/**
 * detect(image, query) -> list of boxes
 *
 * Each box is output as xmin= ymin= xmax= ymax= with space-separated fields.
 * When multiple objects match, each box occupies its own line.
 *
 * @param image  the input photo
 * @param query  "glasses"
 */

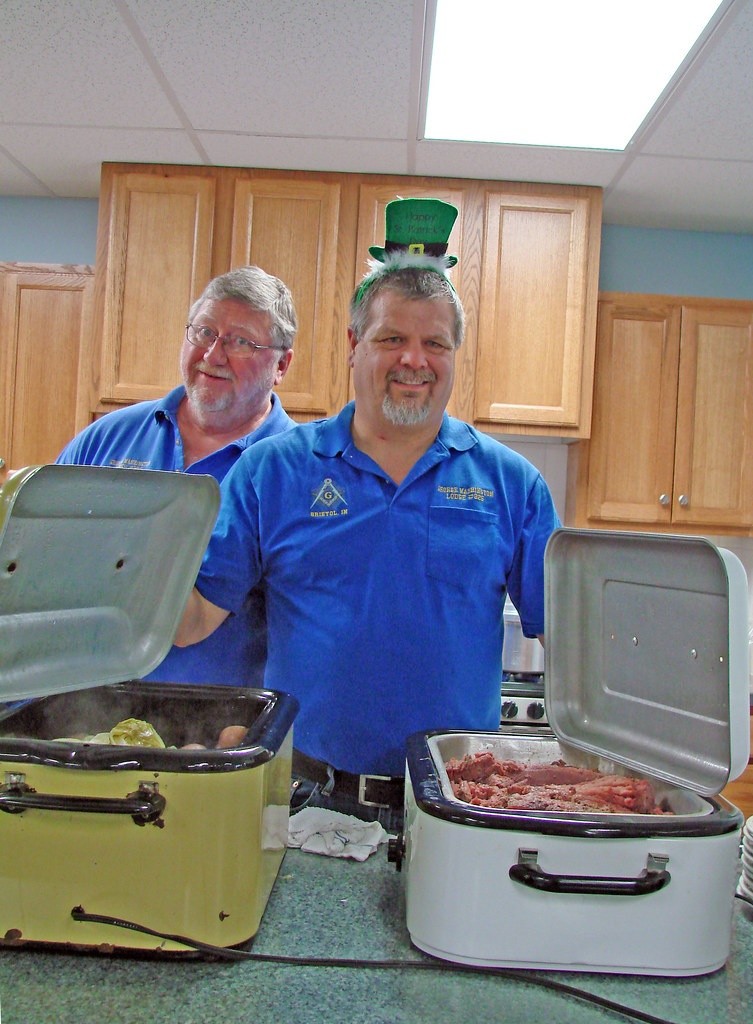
xmin=185 ymin=324 xmax=283 ymax=360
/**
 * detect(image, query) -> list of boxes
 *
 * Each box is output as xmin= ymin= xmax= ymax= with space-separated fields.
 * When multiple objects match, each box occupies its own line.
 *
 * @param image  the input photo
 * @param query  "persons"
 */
xmin=173 ymin=251 xmax=564 ymax=835
xmin=54 ymin=264 xmax=299 ymax=689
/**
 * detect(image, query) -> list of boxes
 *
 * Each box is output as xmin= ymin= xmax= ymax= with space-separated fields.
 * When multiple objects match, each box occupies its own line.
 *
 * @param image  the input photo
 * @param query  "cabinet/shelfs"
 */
xmin=0 ymin=270 xmax=95 ymax=489
xmin=350 ymin=182 xmax=465 ymax=405
xmin=227 ymin=175 xmax=345 ymax=415
xmin=472 ymin=188 xmax=591 ymax=426
xmin=98 ymin=170 xmax=219 ymax=403
xmin=563 ymin=290 xmax=753 ymax=535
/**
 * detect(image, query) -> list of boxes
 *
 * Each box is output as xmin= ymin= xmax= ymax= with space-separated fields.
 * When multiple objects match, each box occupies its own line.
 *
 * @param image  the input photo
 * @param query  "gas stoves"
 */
xmin=500 ymin=676 xmax=549 ymax=724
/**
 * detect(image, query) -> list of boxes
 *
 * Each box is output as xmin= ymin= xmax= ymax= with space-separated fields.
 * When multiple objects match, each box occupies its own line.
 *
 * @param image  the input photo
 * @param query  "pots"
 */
xmin=502 ymin=611 xmax=544 ymax=671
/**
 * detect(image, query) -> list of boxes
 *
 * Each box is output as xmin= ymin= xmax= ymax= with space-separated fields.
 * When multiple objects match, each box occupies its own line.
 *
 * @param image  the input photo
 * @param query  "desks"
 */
xmin=0 ymin=834 xmax=753 ymax=1024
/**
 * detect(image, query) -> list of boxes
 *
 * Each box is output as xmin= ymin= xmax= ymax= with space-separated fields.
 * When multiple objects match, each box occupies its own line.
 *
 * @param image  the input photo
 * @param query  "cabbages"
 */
xmin=110 ymin=718 xmax=165 ymax=748
